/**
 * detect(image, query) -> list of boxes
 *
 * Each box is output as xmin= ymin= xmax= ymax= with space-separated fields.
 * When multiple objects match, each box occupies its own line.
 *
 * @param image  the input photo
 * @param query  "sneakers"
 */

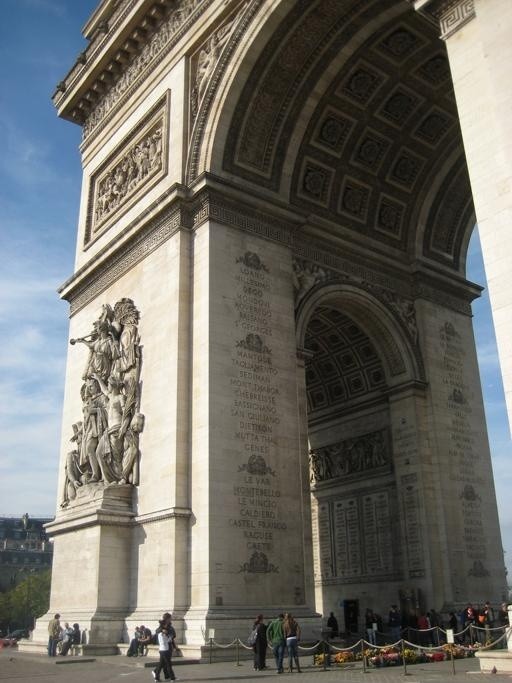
xmin=164 ymin=676 xmax=179 ymax=682
xmin=276 ymin=668 xmax=284 ymax=673
xmin=254 ymin=667 xmax=265 ymax=671
xmin=152 ymin=670 xmax=160 ymax=682
xmin=287 ymin=666 xmax=303 ymax=673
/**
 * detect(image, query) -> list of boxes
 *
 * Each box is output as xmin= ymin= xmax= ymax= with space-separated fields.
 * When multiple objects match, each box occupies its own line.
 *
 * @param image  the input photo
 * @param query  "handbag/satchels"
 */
xmin=478 ymin=616 xmax=485 ymax=623
xmin=373 ymin=623 xmax=378 ymax=631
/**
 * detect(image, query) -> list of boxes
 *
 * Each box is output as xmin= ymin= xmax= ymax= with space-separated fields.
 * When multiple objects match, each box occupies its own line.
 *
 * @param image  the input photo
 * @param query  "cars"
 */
xmin=7 ymin=630 xmax=25 ymax=640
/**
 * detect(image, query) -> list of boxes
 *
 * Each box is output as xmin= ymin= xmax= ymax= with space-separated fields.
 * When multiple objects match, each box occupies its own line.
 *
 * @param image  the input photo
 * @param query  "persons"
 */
xmin=137 ymin=624 xmax=152 ymax=656
xmin=67 ymin=396 xmax=109 ymax=483
xmin=404 ymin=600 xmax=512 ymax=644
xmin=126 ymin=625 xmax=143 ymax=655
xmin=326 ymin=610 xmax=337 ymax=639
xmin=151 ymin=611 xmax=177 ymax=679
xmin=366 ymin=607 xmax=384 ymax=635
xmin=282 ymin=612 xmax=305 ymax=672
xmin=76 ymin=321 xmax=117 ymax=392
xmin=88 ymin=367 xmax=131 ymax=484
xmin=59 ymin=622 xmax=80 ymax=655
xmin=153 ymin=619 xmax=180 ymax=682
xmin=265 ymin=612 xmax=288 ymax=673
xmin=248 ymin=613 xmax=271 ymax=670
xmin=47 ymin=612 xmax=61 ymax=655
xmin=388 ymin=603 xmax=402 ymax=640
xmin=57 ymin=621 xmax=73 ymax=654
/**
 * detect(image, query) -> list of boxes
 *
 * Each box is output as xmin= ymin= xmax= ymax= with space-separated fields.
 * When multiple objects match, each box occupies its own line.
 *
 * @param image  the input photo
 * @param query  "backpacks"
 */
xmin=246 ymin=624 xmax=261 ymax=647
